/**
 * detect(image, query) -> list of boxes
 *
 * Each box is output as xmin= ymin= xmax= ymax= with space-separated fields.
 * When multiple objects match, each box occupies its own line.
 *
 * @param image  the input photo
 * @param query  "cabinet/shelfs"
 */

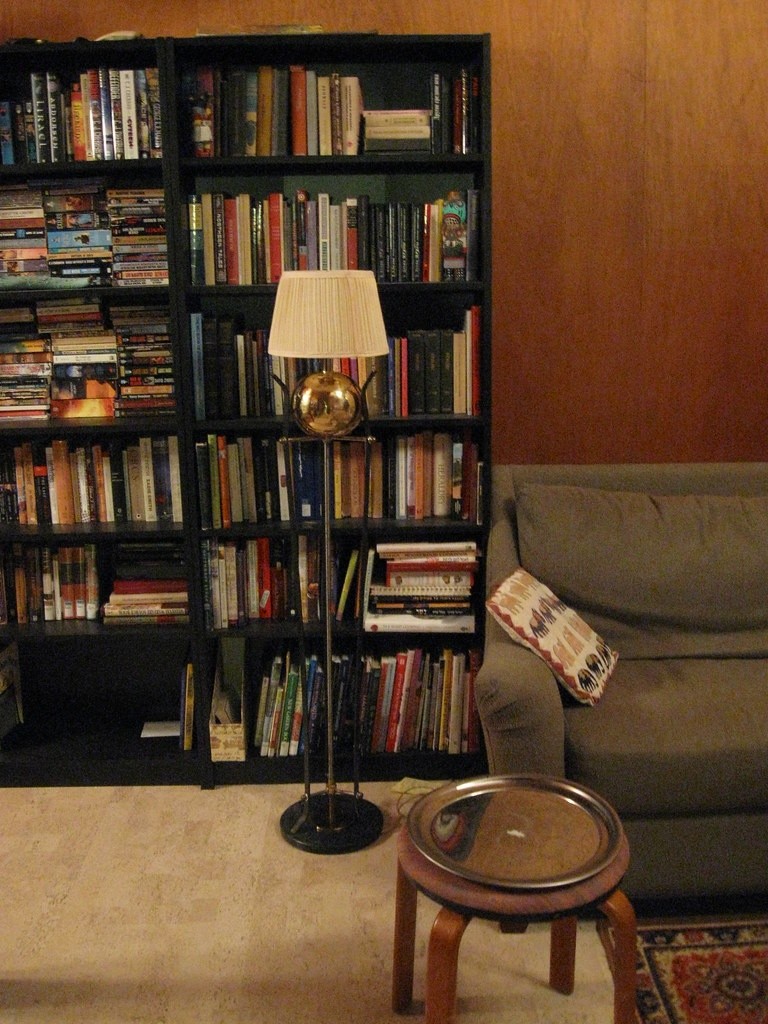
xmin=0 ymin=36 xmax=198 ymax=789
xmin=165 ymin=29 xmax=493 ymax=789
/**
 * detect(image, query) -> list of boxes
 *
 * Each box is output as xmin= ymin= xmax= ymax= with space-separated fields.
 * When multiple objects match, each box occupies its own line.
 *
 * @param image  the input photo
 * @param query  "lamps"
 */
xmin=265 ymin=268 xmax=391 ymax=856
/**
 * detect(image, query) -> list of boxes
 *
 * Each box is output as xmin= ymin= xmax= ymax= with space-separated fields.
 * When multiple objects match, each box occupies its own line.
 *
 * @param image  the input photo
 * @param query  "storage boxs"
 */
xmin=208 ymin=639 xmax=246 ymax=762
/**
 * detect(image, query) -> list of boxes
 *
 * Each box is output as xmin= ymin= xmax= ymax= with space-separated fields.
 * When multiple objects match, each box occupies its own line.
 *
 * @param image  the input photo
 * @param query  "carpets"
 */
xmin=595 ymin=911 xmax=768 ymax=1024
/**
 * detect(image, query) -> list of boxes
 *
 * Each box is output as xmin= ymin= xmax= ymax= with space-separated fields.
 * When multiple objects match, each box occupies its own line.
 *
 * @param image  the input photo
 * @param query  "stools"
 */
xmin=390 ymin=774 xmax=638 ymax=1024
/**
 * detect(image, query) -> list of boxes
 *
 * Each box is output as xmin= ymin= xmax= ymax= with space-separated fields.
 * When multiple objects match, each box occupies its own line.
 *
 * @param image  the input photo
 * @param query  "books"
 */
xmin=1 ymin=642 xmax=481 ymax=759
xmin=0 ymin=66 xmax=480 ymax=633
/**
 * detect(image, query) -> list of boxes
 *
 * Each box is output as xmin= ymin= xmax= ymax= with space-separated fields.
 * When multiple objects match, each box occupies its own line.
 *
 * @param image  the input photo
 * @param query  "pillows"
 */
xmin=489 ymin=566 xmax=619 ymax=705
xmin=514 ymin=482 xmax=768 ymax=657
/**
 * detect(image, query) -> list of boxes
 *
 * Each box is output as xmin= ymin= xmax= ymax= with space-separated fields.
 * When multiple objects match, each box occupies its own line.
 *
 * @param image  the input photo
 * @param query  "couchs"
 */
xmin=475 ymin=465 xmax=768 ymax=930
